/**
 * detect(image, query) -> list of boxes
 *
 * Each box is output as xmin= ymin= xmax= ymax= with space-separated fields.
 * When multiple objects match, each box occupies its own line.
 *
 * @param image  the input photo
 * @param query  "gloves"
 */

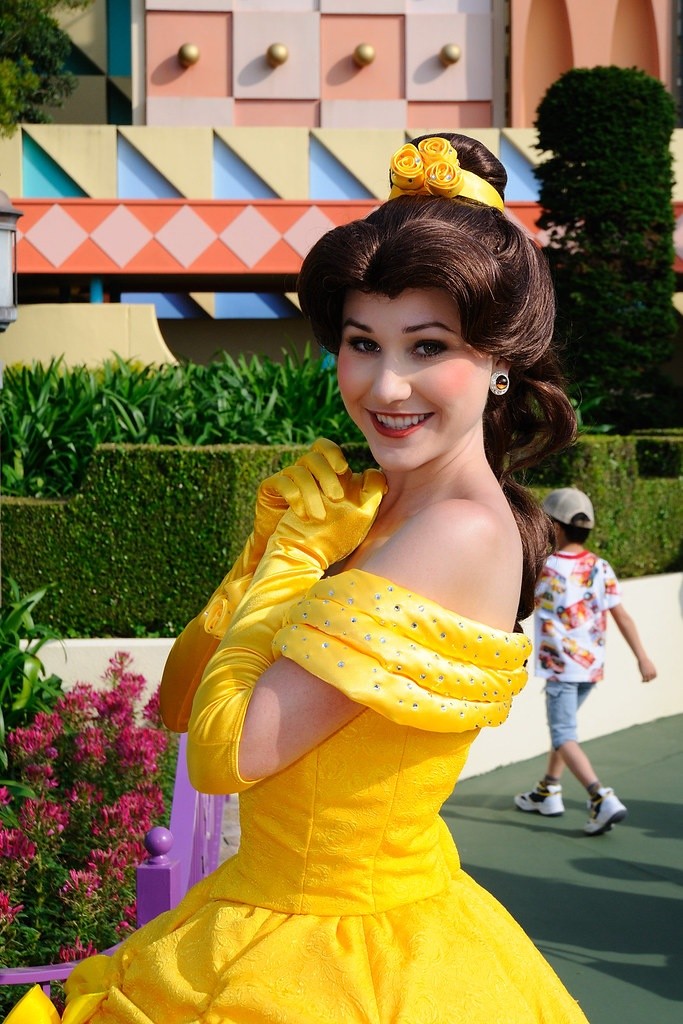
xmin=189 ymin=465 xmax=387 ymax=794
xmin=158 ymin=435 xmax=348 ymax=730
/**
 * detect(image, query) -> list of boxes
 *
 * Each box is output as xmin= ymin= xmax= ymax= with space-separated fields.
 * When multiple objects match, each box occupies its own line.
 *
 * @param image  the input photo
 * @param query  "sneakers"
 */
xmin=512 ymin=781 xmax=566 ymax=816
xmin=583 ymin=786 xmax=627 ymax=836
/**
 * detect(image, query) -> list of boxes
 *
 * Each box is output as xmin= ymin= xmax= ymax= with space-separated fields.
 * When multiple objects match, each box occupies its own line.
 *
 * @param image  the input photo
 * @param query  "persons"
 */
xmin=514 ymin=484 xmax=656 ymax=831
xmin=1 ymin=133 xmax=589 ymax=1024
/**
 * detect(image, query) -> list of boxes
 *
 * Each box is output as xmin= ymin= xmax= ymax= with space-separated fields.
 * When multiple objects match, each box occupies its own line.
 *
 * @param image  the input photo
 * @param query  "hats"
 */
xmin=539 ymin=484 xmax=595 ymax=530
